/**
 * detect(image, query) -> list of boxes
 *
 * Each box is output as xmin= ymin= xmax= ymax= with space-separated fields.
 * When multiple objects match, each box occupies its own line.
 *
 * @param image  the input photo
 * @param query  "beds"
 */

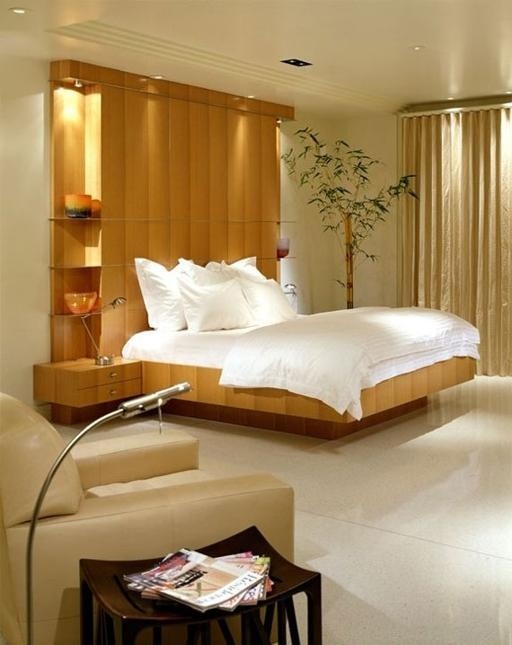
xmin=121 ymin=303 xmax=480 ymax=442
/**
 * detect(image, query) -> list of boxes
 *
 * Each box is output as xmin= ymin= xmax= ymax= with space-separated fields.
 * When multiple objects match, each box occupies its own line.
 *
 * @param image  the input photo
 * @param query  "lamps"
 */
xmin=80 ymin=296 xmax=129 ymax=366
xmin=25 ymin=382 xmax=193 ymax=645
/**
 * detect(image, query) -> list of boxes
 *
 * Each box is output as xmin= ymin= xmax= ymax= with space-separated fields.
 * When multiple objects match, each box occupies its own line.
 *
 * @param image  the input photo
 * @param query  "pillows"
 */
xmin=133 ymin=252 xmax=297 ymax=332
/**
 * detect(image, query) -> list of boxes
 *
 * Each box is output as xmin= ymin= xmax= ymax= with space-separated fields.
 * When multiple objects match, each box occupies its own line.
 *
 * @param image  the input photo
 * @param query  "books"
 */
xmin=121 ymin=546 xmax=276 ymax=614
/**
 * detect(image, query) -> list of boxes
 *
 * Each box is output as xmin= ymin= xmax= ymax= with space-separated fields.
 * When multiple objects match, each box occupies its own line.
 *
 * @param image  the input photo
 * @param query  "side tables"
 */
xmin=76 ymin=524 xmax=321 ymax=645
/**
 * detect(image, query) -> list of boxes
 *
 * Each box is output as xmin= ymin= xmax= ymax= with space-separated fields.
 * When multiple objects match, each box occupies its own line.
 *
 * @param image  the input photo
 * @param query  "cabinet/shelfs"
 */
xmin=51 ymin=215 xmax=102 ymax=318
xmin=277 ymin=219 xmax=299 ymax=295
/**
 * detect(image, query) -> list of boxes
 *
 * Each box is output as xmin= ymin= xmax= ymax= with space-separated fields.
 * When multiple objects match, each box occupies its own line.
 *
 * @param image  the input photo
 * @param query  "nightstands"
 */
xmin=33 ymin=357 xmax=143 ymax=425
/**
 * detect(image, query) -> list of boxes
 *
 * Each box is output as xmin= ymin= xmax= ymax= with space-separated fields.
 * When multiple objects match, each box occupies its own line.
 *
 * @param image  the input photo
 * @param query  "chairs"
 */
xmin=0 ymin=392 xmax=297 ymax=645
